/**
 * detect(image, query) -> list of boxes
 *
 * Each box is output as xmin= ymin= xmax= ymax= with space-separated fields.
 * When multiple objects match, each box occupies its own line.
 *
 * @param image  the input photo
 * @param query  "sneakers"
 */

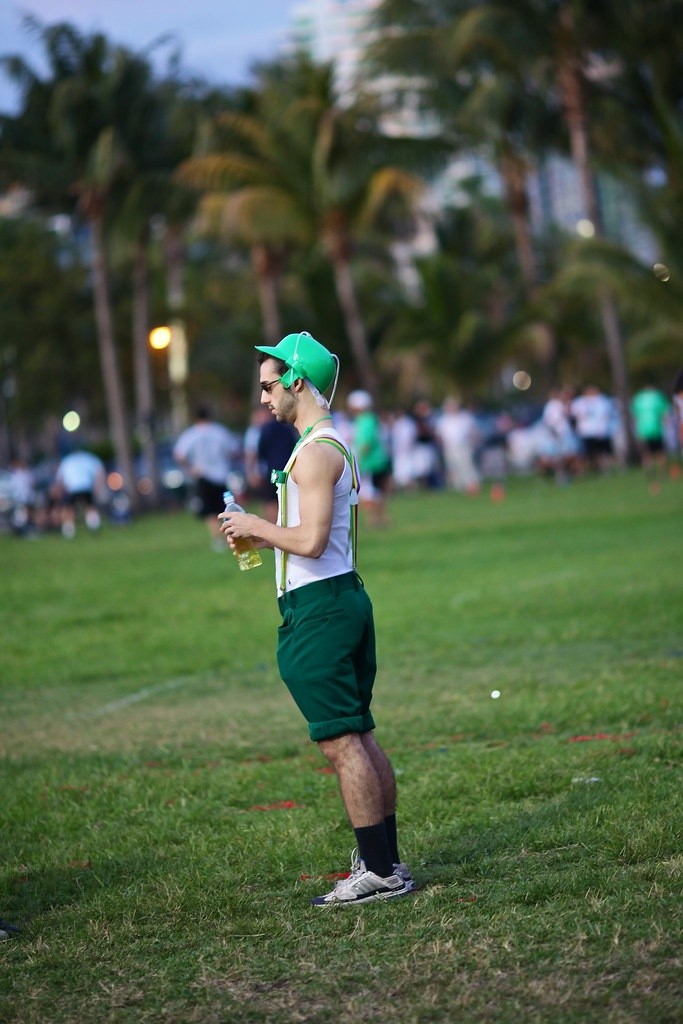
xmin=312 ymin=847 xmax=417 ymax=908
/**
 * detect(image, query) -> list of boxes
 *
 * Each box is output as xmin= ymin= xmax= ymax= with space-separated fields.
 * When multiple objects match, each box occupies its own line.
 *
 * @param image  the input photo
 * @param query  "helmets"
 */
xmin=254 ymin=333 xmax=337 ymax=395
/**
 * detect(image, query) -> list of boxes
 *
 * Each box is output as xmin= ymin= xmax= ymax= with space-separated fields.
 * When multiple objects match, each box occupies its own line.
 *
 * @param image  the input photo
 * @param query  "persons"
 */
xmin=0 ymin=374 xmax=683 ymax=540
xmin=218 ymin=332 xmax=413 ymax=909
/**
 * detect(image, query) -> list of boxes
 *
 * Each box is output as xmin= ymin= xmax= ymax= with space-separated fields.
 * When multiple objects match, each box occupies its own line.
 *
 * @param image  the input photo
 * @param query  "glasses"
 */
xmin=260 ymin=380 xmax=281 ymax=393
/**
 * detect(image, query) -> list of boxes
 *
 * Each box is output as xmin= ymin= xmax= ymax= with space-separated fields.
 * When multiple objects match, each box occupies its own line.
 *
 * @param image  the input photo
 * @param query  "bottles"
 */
xmin=222 ymin=491 xmax=262 ymax=571
